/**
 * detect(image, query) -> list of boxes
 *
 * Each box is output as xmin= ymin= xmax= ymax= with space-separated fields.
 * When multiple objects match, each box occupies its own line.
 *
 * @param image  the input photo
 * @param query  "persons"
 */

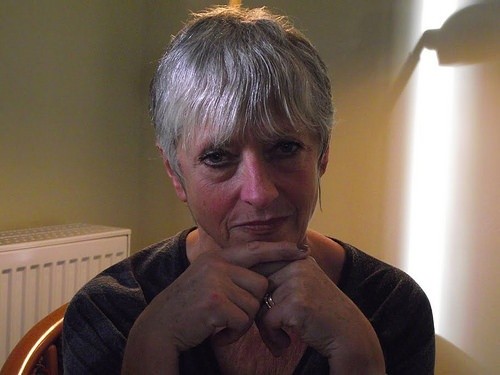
xmin=57 ymin=2 xmax=437 ymax=375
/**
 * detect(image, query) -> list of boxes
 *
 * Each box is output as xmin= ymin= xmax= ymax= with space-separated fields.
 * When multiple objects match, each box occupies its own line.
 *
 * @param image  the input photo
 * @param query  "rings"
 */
xmin=264 ymin=293 xmax=275 ymax=310
